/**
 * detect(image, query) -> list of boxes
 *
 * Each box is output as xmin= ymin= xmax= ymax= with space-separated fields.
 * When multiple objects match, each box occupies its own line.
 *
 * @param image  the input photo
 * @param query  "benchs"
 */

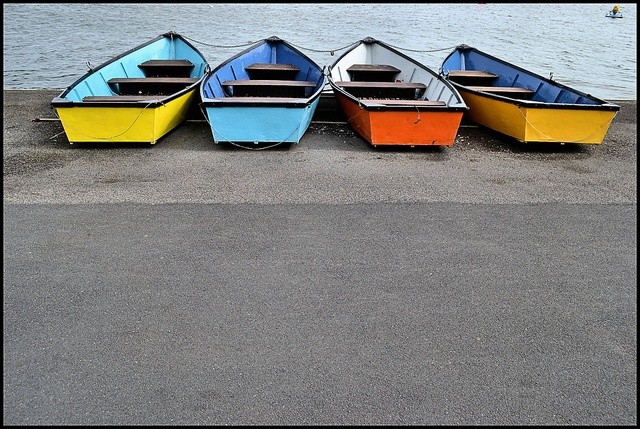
xmin=211 ymin=97 xmax=308 ymax=102
xmin=244 ymin=63 xmax=301 ymax=71
xmin=346 ymin=64 xmax=401 ymax=72
xmin=448 ymin=70 xmax=498 ymax=78
xmin=360 ymin=99 xmax=445 ymax=105
xmin=138 ymin=59 xmax=194 ymax=66
xmin=221 ymin=80 xmax=317 ymax=87
xmin=107 ymin=78 xmax=201 ymax=84
xmin=82 ymin=96 xmax=169 ymax=101
xmin=464 ymin=86 xmax=535 ymax=94
xmin=335 ymin=81 xmax=427 ymax=89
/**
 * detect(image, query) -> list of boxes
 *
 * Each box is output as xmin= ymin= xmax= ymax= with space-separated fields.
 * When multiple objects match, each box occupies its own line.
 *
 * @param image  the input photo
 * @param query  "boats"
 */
xmin=49 ymin=29 xmax=210 ymax=144
xmin=439 ymin=42 xmax=620 ymax=146
xmin=199 ymin=35 xmax=326 ymax=151
xmin=326 ymin=37 xmax=466 ymax=152
